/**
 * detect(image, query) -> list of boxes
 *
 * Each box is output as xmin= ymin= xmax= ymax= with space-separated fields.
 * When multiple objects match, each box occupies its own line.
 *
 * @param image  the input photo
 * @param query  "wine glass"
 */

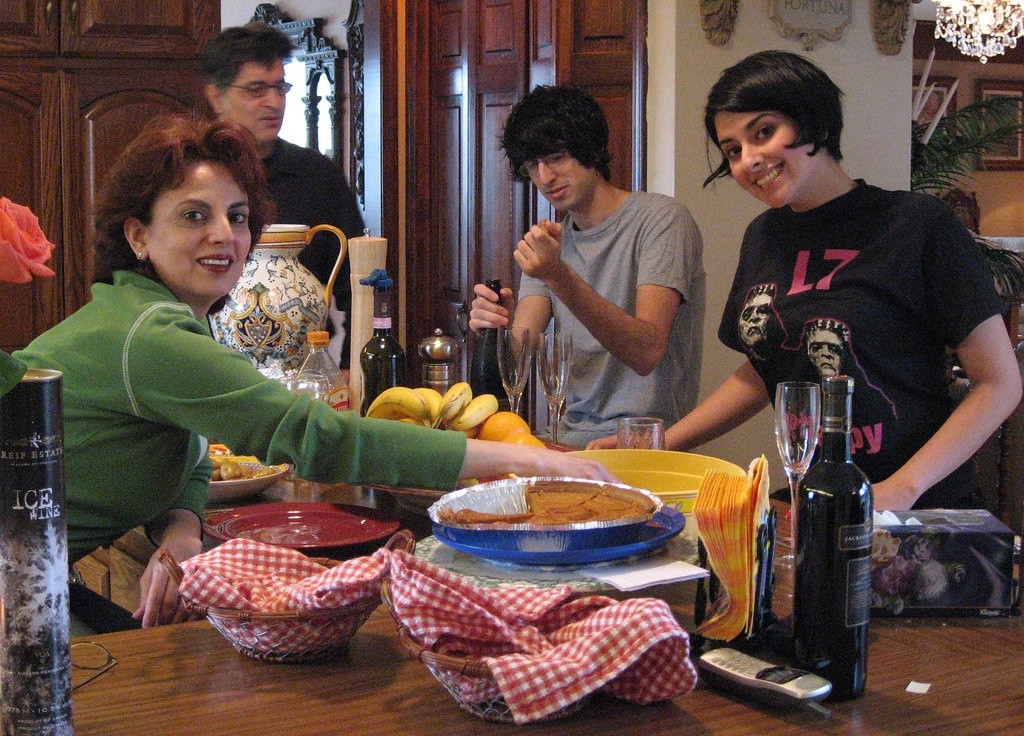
xmin=773 ymin=381 xmax=821 ymax=569
xmin=536 ymin=331 xmax=573 ymax=450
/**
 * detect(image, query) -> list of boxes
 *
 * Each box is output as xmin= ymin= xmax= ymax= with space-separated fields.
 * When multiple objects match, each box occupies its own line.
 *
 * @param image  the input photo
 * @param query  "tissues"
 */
xmin=867 ymin=506 xmax=1023 ymax=618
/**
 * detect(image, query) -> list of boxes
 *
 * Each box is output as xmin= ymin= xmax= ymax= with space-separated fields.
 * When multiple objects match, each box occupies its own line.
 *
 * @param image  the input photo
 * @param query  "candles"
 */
xmin=921 ymin=78 xmax=962 ymax=144
xmin=916 ymin=83 xmax=935 ymax=121
xmin=913 ymin=45 xmax=935 ymax=121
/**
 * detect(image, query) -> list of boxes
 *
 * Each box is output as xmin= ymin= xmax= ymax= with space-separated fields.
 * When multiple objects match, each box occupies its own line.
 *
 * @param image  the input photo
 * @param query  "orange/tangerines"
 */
xmin=480 ymin=412 xmax=547 ymax=449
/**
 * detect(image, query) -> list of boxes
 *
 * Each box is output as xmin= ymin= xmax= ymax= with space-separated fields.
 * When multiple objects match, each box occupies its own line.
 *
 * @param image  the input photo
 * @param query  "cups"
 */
xmin=617 ymin=417 xmax=665 ymax=450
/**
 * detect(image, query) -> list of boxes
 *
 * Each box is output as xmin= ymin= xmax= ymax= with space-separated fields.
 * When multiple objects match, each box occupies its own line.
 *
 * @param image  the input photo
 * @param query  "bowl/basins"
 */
xmin=201 ymin=455 xmax=290 ymax=501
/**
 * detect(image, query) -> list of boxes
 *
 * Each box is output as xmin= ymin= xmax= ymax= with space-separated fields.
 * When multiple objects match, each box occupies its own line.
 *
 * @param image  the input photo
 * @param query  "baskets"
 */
xmin=378 ymin=578 xmax=596 ymax=723
xmin=159 ymin=528 xmax=417 ymax=664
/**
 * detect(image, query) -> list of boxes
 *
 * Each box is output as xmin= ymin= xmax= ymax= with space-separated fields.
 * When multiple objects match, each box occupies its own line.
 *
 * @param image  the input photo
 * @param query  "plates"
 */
xmin=202 ymin=502 xmax=401 ymax=550
xmin=432 ymin=505 xmax=687 ymax=566
xmin=552 ymin=449 xmax=747 ymax=517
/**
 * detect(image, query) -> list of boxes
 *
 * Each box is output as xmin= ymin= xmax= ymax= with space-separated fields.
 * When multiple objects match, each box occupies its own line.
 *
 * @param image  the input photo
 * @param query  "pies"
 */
xmin=434 ymin=481 xmax=654 ymax=524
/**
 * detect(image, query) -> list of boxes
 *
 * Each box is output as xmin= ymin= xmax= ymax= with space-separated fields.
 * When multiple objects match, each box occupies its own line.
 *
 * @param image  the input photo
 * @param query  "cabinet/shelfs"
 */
xmin=0 ymin=0 xmax=222 ymax=348
xmin=379 ymin=0 xmax=645 ymax=403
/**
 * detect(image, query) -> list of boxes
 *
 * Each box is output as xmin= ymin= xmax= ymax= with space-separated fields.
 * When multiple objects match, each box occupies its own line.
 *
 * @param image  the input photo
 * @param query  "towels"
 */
xmin=177 ymin=539 xmax=390 ymax=612
xmin=390 ymin=547 xmax=697 ymax=725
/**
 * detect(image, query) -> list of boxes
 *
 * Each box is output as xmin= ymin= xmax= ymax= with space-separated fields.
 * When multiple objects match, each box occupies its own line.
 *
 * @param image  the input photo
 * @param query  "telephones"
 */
xmin=698 ymin=646 xmax=834 ymax=718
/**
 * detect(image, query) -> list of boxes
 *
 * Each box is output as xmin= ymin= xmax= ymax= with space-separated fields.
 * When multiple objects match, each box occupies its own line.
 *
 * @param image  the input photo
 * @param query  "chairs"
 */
xmin=944 ymin=294 xmax=1024 ymax=533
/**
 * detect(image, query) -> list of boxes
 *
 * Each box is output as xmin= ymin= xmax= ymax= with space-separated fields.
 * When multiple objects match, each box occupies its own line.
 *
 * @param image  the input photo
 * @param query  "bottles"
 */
xmin=790 ymin=374 xmax=874 ymax=703
xmin=0 ymin=368 xmax=75 ymax=736
xmin=496 ymin=326 xmax=532 ymax=415
xmin=417 ymin=327 xmax=461 ymax=397
xmin=469 ymin=279 xmax=524 ymax=413
xmin=293 ymin=330 xmax=352 ymax=500
xmin=359 ymin=268 xmax=406 ymax=498
xmin=207 ymin=222 xmax=347 ymax=382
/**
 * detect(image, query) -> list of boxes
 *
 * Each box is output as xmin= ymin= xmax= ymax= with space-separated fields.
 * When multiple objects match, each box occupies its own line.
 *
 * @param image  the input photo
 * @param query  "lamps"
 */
xmin=933 ymin=0 xmax=1024 ymax=63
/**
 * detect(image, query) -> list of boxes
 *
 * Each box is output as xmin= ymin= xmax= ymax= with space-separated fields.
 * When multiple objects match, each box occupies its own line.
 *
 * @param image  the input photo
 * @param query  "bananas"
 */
xmin=366 ymin=382 xmax=499 ymax=440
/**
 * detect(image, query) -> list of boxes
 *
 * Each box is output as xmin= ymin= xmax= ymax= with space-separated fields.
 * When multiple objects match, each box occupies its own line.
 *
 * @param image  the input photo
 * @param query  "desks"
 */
xmin=68 ymin=432 xmax=1024 ymax=736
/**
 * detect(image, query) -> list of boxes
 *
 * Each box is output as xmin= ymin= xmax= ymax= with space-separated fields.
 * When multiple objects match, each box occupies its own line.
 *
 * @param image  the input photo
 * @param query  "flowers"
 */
xmin=0 ymin=195 xmax=55 ymax=386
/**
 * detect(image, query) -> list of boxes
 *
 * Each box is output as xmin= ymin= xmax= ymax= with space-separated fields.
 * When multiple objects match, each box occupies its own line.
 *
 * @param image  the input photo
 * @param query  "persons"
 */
xmin=10 ymin=115 xmax=617 ymax=639
xmin=201 ymin=24 xmax=371 ymax=370
xmin=586 ymin=51 xmax=1022 ymax=512
xmin=468 ymin=84 xmax=705 ymax=450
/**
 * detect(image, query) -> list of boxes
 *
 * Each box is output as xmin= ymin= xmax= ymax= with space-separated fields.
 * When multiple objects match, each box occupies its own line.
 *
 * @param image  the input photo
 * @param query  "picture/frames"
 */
xmin=974 ymin=80 xmax=1024 ymax=172
xmin=909 ymin=76 xmax=958 ymax=166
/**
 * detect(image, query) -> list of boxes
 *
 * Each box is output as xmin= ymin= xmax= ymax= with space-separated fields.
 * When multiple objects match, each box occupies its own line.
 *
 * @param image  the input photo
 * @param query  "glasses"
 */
xmin=227 ymin=82 xmax=293 ymax=97
xmin=515 ymin=152 xmax=571 ymax=178
